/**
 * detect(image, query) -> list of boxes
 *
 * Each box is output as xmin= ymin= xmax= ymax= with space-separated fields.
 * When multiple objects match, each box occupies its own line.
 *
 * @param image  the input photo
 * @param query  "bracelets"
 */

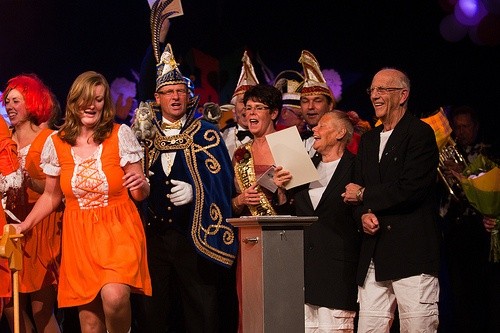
xmin=233 ymin=199 xmax=241 ymax=211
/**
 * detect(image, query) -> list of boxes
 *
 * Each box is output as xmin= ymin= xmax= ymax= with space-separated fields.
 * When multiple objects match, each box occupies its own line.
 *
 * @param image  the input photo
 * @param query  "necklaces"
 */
xmin=305 ymin=139 xmax=316 ymax=154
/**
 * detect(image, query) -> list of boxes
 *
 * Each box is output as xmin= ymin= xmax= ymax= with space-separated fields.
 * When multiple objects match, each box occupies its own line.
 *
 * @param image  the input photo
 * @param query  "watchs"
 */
xmin=356 ymin=187 xmax=365 ymax=203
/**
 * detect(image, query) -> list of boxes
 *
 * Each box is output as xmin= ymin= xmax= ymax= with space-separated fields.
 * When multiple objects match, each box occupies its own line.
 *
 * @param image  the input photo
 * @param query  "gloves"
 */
xmin=169 ymin=179 xmax=193 ymax=206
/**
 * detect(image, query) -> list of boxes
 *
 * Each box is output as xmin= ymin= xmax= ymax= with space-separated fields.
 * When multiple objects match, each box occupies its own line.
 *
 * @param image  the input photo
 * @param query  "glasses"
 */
xmin=366 ymin=87 xmax=403 ymax=95
xmin=242 ymin=105 xmax=271 ymax=113
xmin=288 ymin=108 xmax=303 ymax=119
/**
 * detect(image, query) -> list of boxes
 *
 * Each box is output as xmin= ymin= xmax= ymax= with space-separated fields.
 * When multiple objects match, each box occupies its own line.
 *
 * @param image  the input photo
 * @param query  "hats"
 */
xmin=273 ymin=70 xmax=306 ymax=108
xmin=230 ymin=51 xmax=260 ymax=103
xmin=298 ymin=50 xmax=337 ymax=104
xmin=156 ymin=43 xmax=187 ymax=91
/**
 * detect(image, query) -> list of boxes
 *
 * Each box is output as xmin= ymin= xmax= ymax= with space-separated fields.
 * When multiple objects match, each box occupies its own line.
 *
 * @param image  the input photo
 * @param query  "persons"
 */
xmin=341 ymin=67 xmax=442 ymax=333
xmin=3 ymin=70 xmax=151 ymax=333
xmin=129 ymin=76 xmax=240 ymax=333
xmin=2 ymin=73 xmax=65 ymax=333
xmin=218 ymin=79 xmax=371 ymax=161
xmin=0 ymin=113 xmax=36 ymax=333
xmin=482 ymin=215 xmax=497 ymax=233
xmin=231 ymin=85 xmax=293 ymax=333
xmin=272 ymin=109 xmax=363 ymax=333
xmin=441 ymin=109 xmax=500 ymax=333
